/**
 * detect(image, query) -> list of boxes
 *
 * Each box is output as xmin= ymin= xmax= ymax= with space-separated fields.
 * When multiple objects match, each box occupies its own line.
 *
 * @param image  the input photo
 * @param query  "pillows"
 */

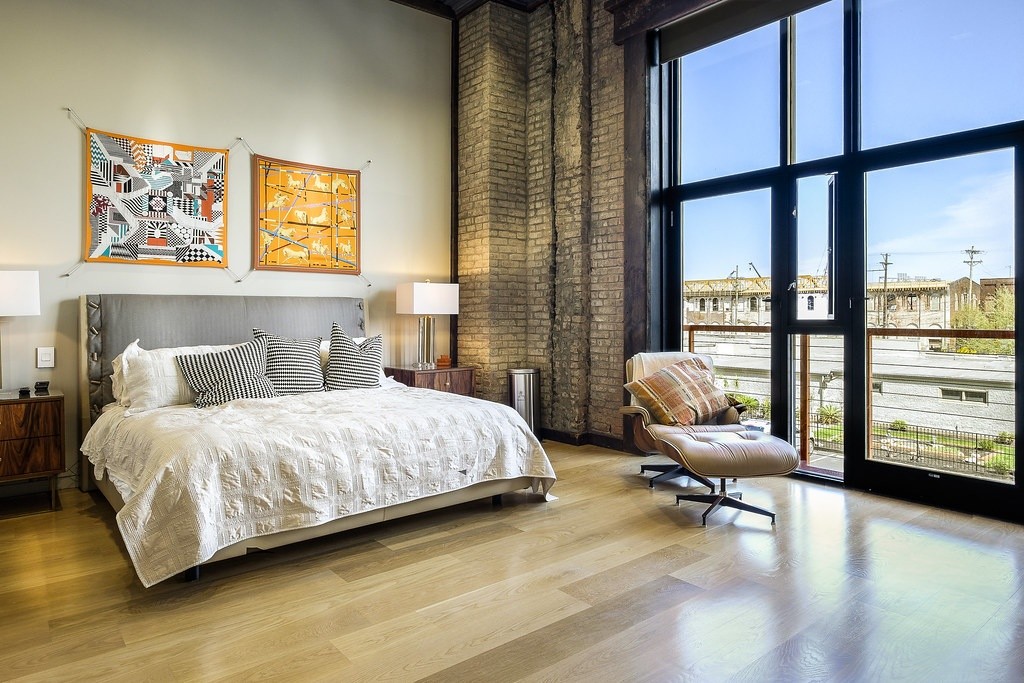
xmin=175 ymin=336 xmax=281 ymax=408
xmin=622 ymin=357 xmax=729 ymax=428
xmin=252 ymin=327 xmax=325 ymax=397
xmin=320 ymin=337 xmax=388 ymax=381
xmin=121 ymin=338 xmax=250 ymax=417
xmin=324 ymin=321 xmax=383 ymax=391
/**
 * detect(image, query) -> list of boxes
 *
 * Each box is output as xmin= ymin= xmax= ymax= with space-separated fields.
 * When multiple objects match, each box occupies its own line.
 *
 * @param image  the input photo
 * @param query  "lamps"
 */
xmin=395 ymin=279 xmax=459 ymax=367
xmin=0 ymin=270 xmax=41 ymax=390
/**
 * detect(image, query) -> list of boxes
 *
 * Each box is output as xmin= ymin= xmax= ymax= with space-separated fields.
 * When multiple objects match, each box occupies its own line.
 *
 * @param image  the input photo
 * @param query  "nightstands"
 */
xmin=0 ymin=389 xmax=66 ymax=515
xmin=385 ymin=367 xmax=476 ymax=398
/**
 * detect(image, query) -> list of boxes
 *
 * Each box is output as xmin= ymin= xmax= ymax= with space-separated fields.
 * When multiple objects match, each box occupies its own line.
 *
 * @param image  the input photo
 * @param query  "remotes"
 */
xmin=18 ymin=387 xmax=30 ymax=393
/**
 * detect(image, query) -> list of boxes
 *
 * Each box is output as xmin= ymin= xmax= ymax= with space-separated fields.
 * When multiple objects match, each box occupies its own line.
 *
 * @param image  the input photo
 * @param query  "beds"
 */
xmin=78 ymin=295 xmax=560 ymax=585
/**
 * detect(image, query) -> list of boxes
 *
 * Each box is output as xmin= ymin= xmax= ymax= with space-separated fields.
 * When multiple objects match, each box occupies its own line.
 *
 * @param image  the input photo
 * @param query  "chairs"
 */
xmin=619 ymin=351 xmax=800 ymax=527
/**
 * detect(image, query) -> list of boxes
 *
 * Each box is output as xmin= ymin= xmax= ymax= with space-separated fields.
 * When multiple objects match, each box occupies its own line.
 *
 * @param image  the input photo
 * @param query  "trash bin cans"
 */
xmin=507 ymin=367 xmax=542 ymax=441
